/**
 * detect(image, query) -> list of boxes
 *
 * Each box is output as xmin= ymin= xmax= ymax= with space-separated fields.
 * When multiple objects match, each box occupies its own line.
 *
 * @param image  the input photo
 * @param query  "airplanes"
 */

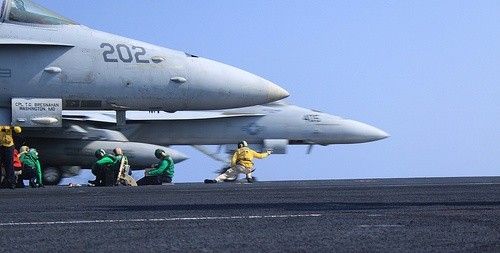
xmin=62 ymin=102 xmax=390 ymax=182
xmin=0 ymin=0 xmax=290 ymax=188
xmin=16 ymin=120 xmax=189 ymax=184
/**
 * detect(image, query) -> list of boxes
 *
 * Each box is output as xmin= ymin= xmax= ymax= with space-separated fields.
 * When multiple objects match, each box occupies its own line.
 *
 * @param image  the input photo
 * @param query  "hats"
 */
xmin=19 ymin=144 xmax=30 ymax=154
xmin=152 ymin=148 xmax=165 ymax=158
xmin=112 ymin=147 xmax=125 ymax=155
xmin=93 ymin=149 xmax=106 ymax=159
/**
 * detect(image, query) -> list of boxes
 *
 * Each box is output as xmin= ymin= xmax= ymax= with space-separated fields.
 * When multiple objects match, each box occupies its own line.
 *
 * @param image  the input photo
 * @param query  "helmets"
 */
xmin=236 ymin=140 xmax=250 ymax=149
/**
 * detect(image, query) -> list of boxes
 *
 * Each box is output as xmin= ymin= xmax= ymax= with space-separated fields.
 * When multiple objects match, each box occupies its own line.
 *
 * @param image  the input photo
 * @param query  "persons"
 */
xmin=204 ymin=140 xmax=274 ymax=183
xmin=0 ymin=146 xmax=45 ymax=189
xmin=87 ymin=147 xmax=133 ymax=186
xmin=136 ymin=149 xmax=175 ymax=186
xmin=0 ymin=126 xmax=22 ymax=190
